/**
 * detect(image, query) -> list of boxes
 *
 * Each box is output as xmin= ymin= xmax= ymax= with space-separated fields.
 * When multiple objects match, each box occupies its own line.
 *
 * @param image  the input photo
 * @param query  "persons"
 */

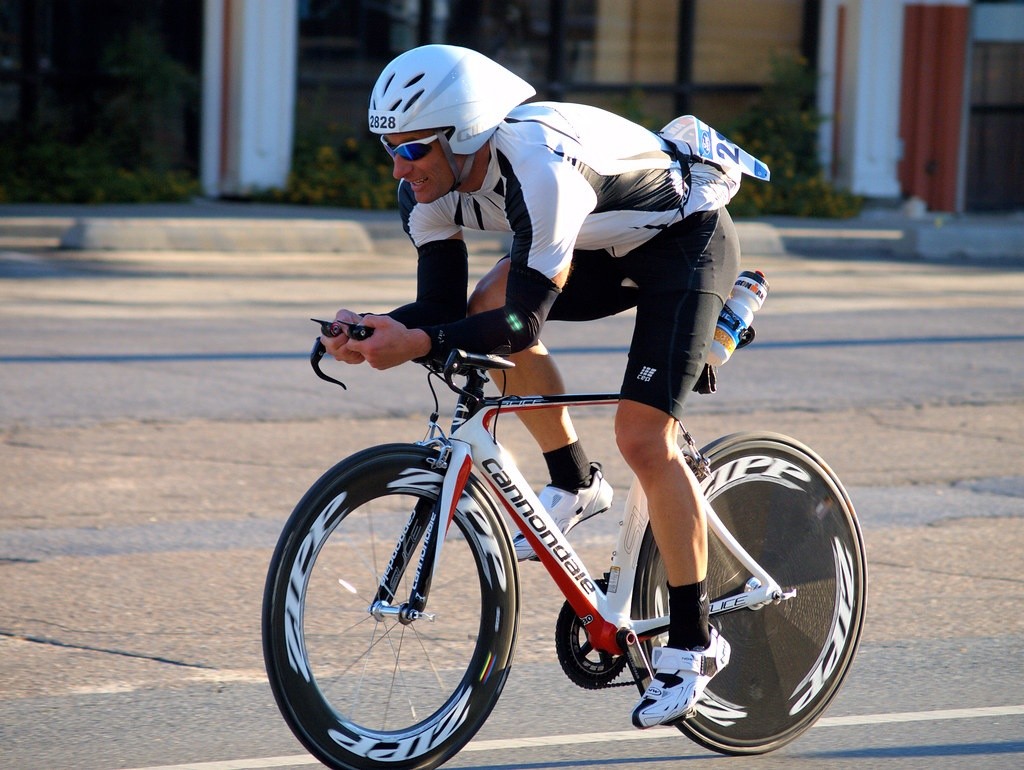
xmin=321 ymin=46 xmax=742 ymax=728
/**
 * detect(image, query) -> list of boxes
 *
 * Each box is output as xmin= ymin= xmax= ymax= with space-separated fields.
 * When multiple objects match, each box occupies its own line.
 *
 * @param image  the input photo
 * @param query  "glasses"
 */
xmin=381 ymin=129 xmax=453 ymax=162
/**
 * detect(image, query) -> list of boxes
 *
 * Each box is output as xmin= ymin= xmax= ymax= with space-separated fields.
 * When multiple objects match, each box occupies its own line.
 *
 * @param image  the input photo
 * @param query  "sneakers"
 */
xmin=512 ymin=462 xmax=613 ymax=562
xmin=630 ymin=623 xmax=731 ymax=729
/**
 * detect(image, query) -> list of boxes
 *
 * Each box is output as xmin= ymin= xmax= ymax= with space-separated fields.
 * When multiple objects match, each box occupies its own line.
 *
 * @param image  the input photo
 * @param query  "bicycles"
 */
xmin=263 ymin=316 xmax=869 ymax=770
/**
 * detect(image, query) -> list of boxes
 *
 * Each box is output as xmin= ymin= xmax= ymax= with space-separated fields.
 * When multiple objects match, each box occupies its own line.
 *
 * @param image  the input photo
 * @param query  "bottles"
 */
xmin=704 ymin=269 xmax=770 ymax=368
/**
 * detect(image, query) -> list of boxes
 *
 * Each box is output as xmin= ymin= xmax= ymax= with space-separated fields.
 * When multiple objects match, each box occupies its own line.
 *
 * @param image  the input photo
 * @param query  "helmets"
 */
xmin=368 ymin=44 xmax=536 ymax=156
